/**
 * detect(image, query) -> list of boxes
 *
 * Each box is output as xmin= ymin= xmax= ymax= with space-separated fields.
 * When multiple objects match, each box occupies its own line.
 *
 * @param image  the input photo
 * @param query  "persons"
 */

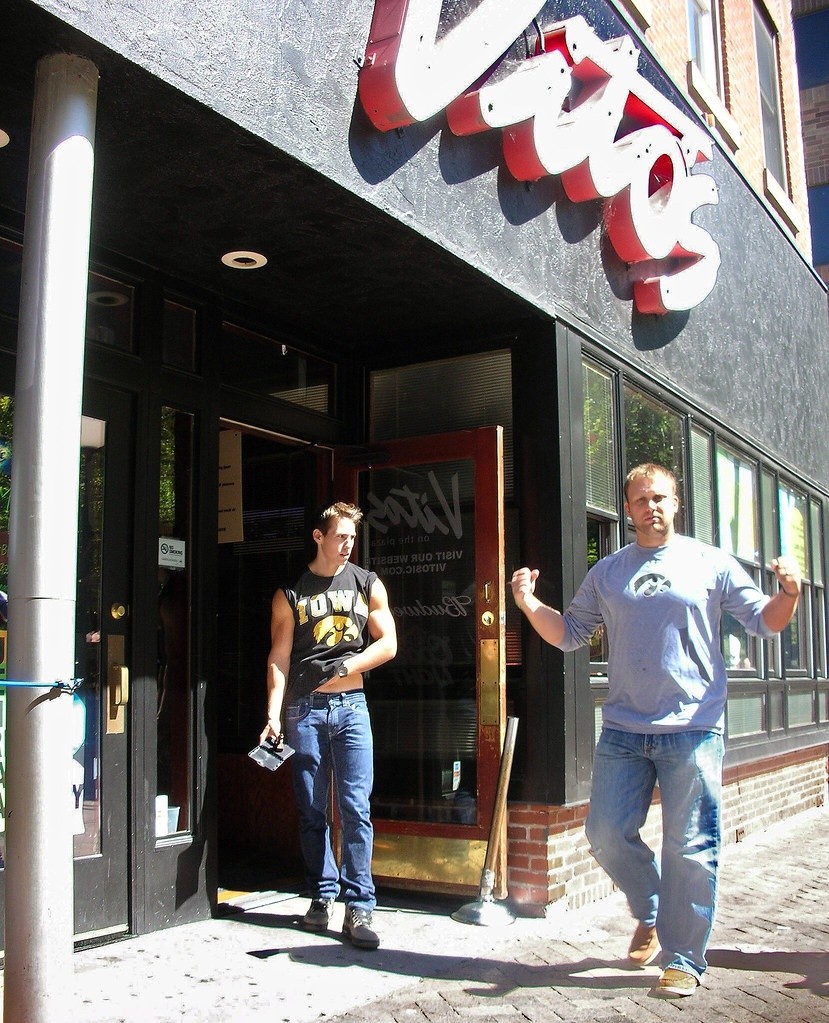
xmin=512 ymin=464 xmax=801 ymax=995
xmin=157 ymin=545 xmax=187 ymax=830
xmin=259 ymin=501 xmax=397 ymax=947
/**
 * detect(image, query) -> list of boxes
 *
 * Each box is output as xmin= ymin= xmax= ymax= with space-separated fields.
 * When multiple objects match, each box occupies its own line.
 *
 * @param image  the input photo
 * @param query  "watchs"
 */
xmin=338 ymin=662 xmax=348 ymax=678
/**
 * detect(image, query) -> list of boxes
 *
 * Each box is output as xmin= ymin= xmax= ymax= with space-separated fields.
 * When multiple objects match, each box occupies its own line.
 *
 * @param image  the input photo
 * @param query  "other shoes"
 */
xmin=303 ymin=898 xmax=333 ymax=931
xmin=656 ymin=969 xmax=697 ymax=995
xmin=343 ymin=907 xmax=379 ymax=949
xmin=630 ymin=927 xmax=660 ymax=966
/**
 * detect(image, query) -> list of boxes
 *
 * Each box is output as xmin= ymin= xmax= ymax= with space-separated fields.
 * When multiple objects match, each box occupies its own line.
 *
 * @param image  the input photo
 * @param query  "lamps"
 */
xmin=220 ymin=248 xmax=269 ymax=270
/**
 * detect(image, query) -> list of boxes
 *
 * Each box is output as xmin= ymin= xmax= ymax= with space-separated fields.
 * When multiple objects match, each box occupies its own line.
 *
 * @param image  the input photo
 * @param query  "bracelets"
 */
xmin=783 ymin=588 xmax=800 ymax=596
